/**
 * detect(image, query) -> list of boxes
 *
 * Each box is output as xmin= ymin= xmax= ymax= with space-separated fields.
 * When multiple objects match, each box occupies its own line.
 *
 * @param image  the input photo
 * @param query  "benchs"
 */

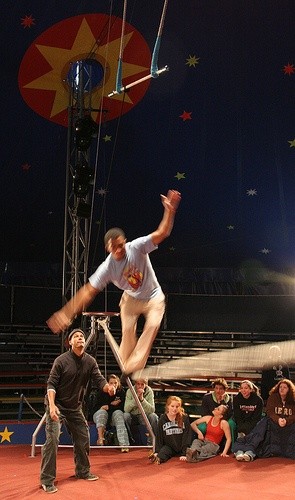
xmin=0 ymin=324 xmax=295 ymax=419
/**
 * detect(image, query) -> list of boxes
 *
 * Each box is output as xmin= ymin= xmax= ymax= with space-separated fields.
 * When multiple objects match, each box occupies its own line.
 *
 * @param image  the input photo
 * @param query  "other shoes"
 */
xmin=75 ymin=473 xmax=99 ymax=480
xmin=41 ymin=483 xmax=58 ymax=493
xmin=120 ymin=448 xmax=129 ymax=453
xmin=96 ymin=439 xmax=104 ymax=446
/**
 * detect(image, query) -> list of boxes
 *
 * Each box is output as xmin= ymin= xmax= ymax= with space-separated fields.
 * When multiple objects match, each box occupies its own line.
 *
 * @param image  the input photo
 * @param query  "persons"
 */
xmin=123 ymin=377 xmax=158 ymax=449
xmin=186 ymin=405 xmax=232 ymax=463
xmin=148 ymin=395 xmax=192 ymax=465
xmin=264 ymin=379 xmax=295 ymax=459
xmin=46 ymin=190 xmax=181 ymax=368
xmin=92 ymin=374 xmax=130 ymax=452
xmin=234 ymin=380 xmax=263 ymax=462
xmin=196 ymin=378 xmax=235 ymax=454
xmin=40 ymin=329 xmax=116 ymax=494
xmin=260 ymin=347 xmax=290 ymax=408
